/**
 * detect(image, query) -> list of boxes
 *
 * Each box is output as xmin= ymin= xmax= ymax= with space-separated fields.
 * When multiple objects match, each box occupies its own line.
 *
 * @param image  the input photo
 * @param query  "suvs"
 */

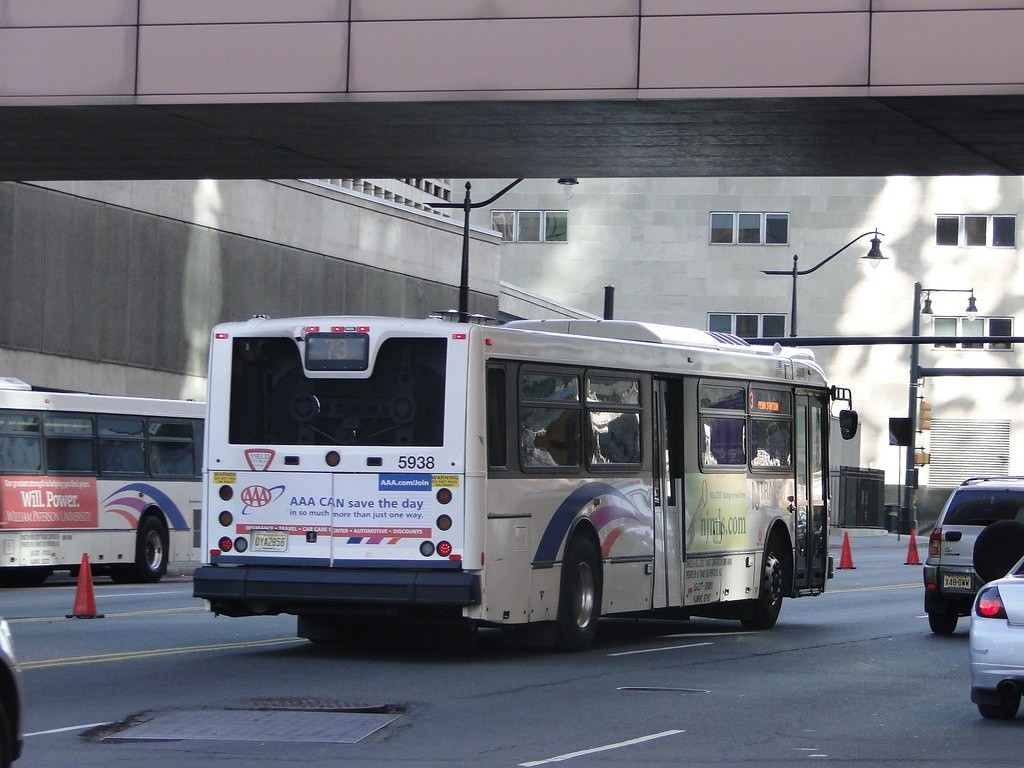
xmin=923 ymin=476 xmax=1024 ymax=634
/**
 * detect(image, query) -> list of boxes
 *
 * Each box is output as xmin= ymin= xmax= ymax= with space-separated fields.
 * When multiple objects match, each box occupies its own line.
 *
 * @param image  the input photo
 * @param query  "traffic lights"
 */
xmin=920 ymin=402 xmax=932 ymax=430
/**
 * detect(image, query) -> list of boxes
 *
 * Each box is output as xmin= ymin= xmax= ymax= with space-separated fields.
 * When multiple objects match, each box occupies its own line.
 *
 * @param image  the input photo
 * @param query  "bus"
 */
xmin=195 ymin=316 xmax=858 ymax=652
xmin=0 ymin=377 xmax=207 ymax=584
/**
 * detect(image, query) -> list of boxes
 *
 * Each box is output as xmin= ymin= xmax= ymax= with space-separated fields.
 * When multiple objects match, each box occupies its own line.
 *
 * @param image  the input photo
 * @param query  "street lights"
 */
xmin=422 ymin=177 xmax=580 ymax=326
xmin=900 ymin=281 xmax=978 ymax=536
xmin=765 ymin=230 xmax=890 ymax=348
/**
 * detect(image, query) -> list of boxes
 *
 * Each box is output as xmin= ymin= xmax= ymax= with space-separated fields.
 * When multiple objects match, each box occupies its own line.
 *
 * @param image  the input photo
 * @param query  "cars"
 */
xmin=968 ymin=556 xmax=1024 ymax=720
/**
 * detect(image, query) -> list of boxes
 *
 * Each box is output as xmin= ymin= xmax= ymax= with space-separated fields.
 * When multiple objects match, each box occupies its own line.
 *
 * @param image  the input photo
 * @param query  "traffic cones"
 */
xmin=65 ymin=553 xmax=105 ymax=619
xmin=903 ymin=529 xmax=923 ymax=565
xmin=836 ymin=532 xmax=856 ymax=569
